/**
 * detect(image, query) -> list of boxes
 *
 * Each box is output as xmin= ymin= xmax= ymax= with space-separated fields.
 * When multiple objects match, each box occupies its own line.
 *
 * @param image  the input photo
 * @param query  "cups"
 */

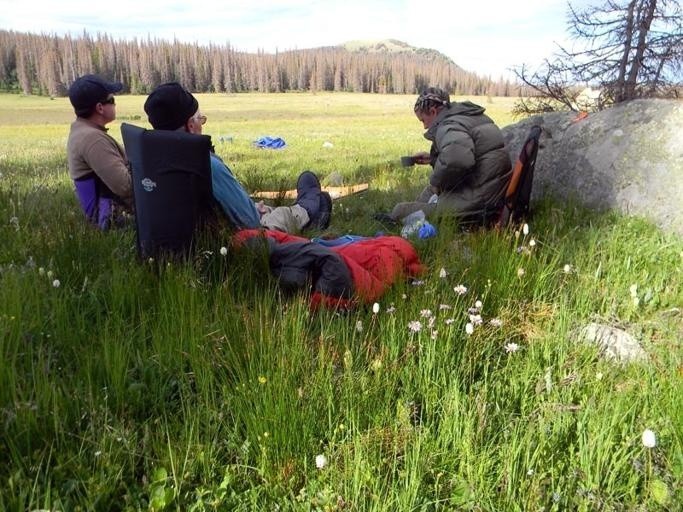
xmin=401 ymin=156 xmax=416 ymax=167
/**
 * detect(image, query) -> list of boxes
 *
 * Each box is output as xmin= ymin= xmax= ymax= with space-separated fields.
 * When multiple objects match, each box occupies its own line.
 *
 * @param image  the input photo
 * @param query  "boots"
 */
xmin=292 ymin=170 xmax=321 ymax=221
xmin=301 ymin=190 xmax=332 ymax=234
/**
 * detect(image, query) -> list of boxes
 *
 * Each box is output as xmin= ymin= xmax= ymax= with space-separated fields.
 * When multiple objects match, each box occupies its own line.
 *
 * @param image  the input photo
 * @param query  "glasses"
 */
xmin=101 ymin=97 xmax=114 ymax=106
xmin=196 ymin=115 xmax=206 ymax=126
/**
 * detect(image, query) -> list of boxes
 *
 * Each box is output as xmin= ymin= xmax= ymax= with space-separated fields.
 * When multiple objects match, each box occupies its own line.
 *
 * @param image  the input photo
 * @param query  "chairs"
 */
xmin=120 ymin=122 xmax=213 ymax=270
xmin=430 ymin=125 xmax=541 ymax=230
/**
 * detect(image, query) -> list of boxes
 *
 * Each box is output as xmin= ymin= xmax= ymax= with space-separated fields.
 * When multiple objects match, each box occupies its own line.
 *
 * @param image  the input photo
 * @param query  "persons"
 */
xmin=391 ymin=87 xmax=514 ymax=225
xmin=139 ymin=81 xmax=332 ymax=236
xmin=66 ymin=76 xmax=135 ymax=227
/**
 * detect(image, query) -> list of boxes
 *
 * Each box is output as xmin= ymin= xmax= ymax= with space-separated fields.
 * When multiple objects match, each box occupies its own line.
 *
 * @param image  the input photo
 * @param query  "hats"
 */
xmin=67 ymin=74 xmax=122 ymax=109
xmin=143 ymin=81 xmax=199 ymax=131
